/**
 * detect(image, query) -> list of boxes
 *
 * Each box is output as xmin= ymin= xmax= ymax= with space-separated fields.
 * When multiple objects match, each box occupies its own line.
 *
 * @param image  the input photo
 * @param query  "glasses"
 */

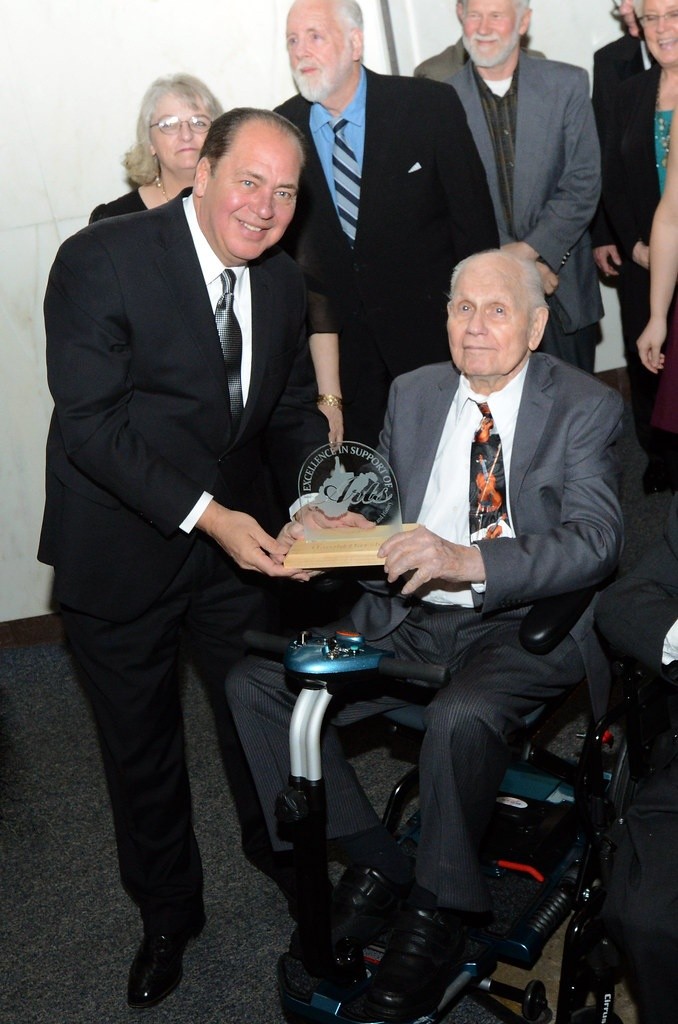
xmin=150 ymin=114 xmax=212 ymax=135
xmin=638 ymin=10 xmax=678 ymax=26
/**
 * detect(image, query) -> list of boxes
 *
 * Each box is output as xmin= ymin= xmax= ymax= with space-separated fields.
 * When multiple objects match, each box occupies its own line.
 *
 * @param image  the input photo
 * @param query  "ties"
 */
xmin=470 ymin=401 xmax=513 ymax=540
xmin=215 ymin=270 xmax=244 ymax=439
xmin=327 ymin=118 xmax=360 ymax=250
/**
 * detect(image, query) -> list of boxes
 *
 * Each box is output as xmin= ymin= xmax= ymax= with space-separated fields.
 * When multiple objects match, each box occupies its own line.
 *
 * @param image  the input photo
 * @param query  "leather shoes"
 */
xmin=290 ymin=857 xmax=414 ymax=958
xmin=127 ymin=912 xmax=208 ymax=1007
xmin=365 ymin=906 xmax=459 ymax=1020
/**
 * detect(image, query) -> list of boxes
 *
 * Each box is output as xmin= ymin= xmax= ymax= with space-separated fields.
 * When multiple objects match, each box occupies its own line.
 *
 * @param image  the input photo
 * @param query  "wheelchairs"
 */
xmin=237 ymin=569 xmax=670 ymax=1024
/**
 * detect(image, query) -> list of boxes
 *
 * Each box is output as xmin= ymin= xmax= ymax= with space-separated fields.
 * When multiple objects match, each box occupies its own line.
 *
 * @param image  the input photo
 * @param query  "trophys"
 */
xmin=280 ymin=439 xmax=422 ymax=569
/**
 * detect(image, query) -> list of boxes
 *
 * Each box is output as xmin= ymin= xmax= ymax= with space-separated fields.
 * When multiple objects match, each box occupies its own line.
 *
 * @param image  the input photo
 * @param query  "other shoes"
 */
xmin=642 ymin=462 xmax=669 ymax=496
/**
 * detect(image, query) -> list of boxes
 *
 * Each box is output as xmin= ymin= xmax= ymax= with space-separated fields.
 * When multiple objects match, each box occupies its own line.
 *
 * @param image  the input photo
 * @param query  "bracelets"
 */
xmin=317 ymin=394 xmax=344 ymax=413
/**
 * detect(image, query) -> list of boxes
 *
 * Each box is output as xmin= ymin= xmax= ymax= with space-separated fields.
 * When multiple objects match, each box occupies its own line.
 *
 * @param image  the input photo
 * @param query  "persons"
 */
xmin=595 ymin=520 xmax=677 ymax=1024
xmin=226 ymin=250 xmax=623 ymax=1018
xmin=43 ymin=107 xmax=372 ymax=1009
xmin=84 ymin=72 xmax=352 ymax=456
xmin=416 ymin=0 xmax=678 ymax=493
xmin=258 ymin=2 xmax=504 ymax=448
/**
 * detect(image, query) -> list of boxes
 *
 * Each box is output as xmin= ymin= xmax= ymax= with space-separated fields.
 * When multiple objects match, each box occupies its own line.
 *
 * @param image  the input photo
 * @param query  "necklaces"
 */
xmin=656 ymin=85 xmax=673 ymax=167
xmin=156 ymin=179 xmax=171 ymax=202
